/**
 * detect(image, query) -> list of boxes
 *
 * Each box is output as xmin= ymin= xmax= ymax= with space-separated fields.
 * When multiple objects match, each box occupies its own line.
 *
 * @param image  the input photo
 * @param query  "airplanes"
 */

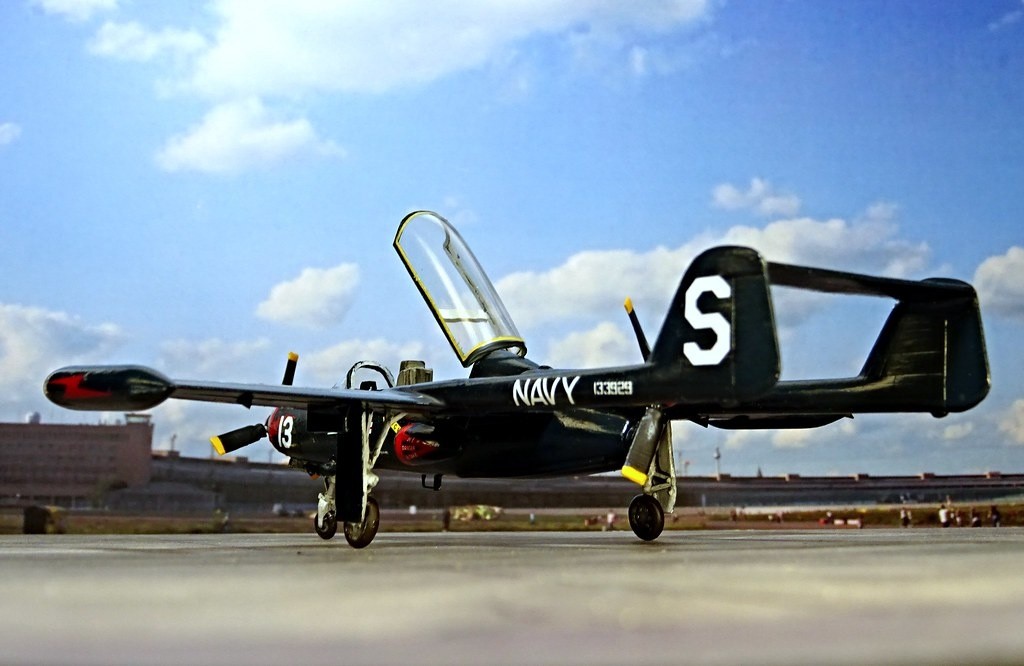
xmin=45 ymin=208 xmax=990 ymax=549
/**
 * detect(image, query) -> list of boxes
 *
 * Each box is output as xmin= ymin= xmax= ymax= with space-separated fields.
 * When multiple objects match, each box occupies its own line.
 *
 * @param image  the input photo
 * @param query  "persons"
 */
xmin=938 ymin=502 xmax=982 ymax=527
xmin=442 ymin=508 xmax=450 ymax=532
xmin=768 ymin=514 xmax=781 ymax=523
xmin=731 ymin=508 xmax=747 ymax=521
xmin=991 ymin=505 xmax=1001 ymax=527
xmin=825 ymin=511 xmax=834 ymax=524
xmin=900 ymin=508 xmax=913 ymax=528
xmin=607 ymin=509 xmax=615 ymax=531
